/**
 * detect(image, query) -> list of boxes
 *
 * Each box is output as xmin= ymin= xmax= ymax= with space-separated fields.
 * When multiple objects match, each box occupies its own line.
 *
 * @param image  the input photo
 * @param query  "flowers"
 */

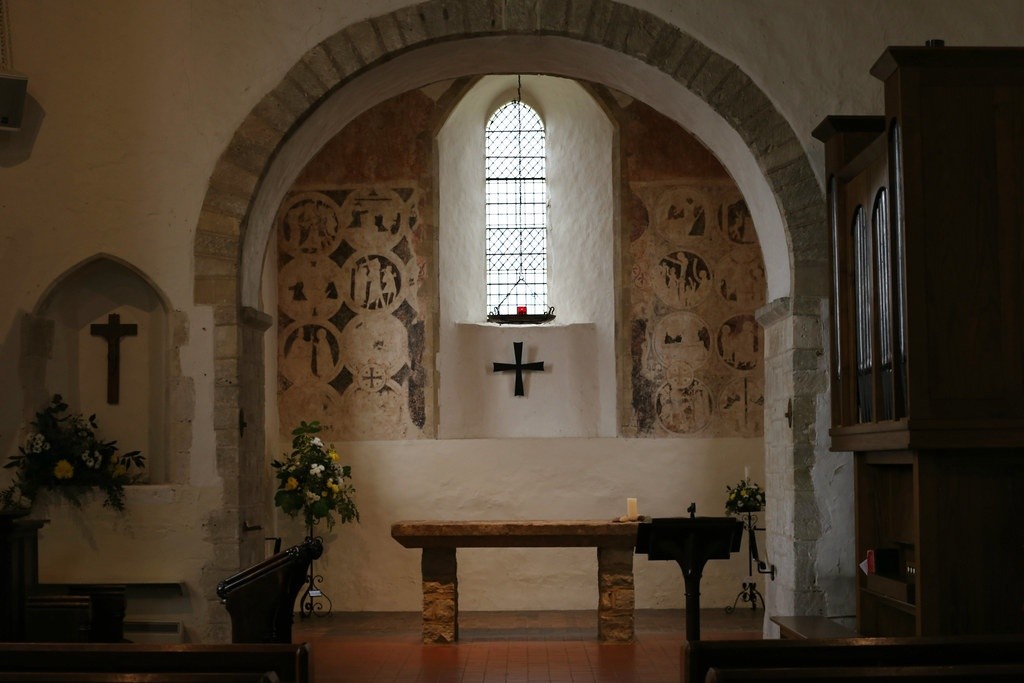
xmin=267 ymin=418 xmax=365 ymax=534
xmin=0 ymin=393 xmax=150 ymax=525
xmin=724 ymin=474 xmax=768 ymax=518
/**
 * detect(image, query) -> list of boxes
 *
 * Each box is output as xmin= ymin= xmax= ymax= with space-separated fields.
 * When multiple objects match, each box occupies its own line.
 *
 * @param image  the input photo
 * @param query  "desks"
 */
xmin=388 ymin=518 xmax=655 ymax=645
xmin=649 ymin=517 xmax=744 ymax=638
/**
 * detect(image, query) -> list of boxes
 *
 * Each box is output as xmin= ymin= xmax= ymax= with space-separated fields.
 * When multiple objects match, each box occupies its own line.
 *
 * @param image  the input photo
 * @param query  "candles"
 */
xmin=624 ymin=497 xmax=640 ymax=520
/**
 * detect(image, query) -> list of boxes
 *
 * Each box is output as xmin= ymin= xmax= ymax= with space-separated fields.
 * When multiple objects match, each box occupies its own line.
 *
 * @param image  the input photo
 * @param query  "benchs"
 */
xmin=676 ymin=613 xmax=1024 ymax=683
xmin=0 ymin=641 xmax=312 ymax=683
